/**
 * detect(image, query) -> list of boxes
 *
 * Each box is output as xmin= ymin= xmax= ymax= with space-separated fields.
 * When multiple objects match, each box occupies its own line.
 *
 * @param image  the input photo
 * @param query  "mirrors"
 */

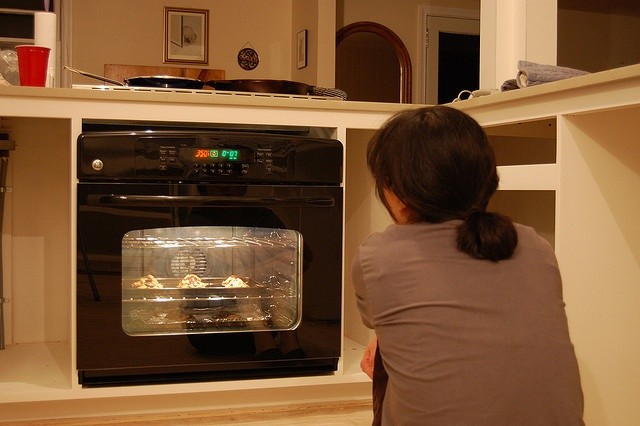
xmin=336 ymin=20 xmax=413 ymax=104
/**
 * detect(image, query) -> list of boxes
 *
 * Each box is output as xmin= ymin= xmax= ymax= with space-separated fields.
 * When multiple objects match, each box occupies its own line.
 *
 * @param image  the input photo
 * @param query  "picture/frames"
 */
xmin=296 ymin=29 xmax=308 ymax=68
xmin=162 ymin=5 xmax=211 ymax=67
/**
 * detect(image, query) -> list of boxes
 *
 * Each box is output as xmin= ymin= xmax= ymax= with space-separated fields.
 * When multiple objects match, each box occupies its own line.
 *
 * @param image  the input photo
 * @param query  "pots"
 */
xmin=62 ymin=65 xmax=205 ymax=89
xmin=206 ymin=78 xmax=347 ymax=101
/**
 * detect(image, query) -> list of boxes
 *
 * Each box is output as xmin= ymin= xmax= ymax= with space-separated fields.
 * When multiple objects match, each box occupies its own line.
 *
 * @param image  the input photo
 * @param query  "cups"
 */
xmin=15 ymin=45 xmax=51 ymax=87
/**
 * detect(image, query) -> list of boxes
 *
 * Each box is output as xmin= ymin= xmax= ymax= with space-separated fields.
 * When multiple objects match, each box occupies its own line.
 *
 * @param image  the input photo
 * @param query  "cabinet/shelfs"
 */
xmin=439 ymin=65 xmax=640 ymax=425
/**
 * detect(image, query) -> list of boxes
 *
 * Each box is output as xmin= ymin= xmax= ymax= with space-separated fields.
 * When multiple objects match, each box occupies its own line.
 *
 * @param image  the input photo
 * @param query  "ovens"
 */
xmin=75 ymin=129 xmax=344 ymax=389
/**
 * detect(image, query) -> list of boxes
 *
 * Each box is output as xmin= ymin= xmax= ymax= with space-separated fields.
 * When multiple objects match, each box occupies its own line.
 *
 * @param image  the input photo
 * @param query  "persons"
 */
xmin=348 ymin=106 xmax=585 ymax=425
xmin=179 ymin=148 xmax=313 ymax=376
